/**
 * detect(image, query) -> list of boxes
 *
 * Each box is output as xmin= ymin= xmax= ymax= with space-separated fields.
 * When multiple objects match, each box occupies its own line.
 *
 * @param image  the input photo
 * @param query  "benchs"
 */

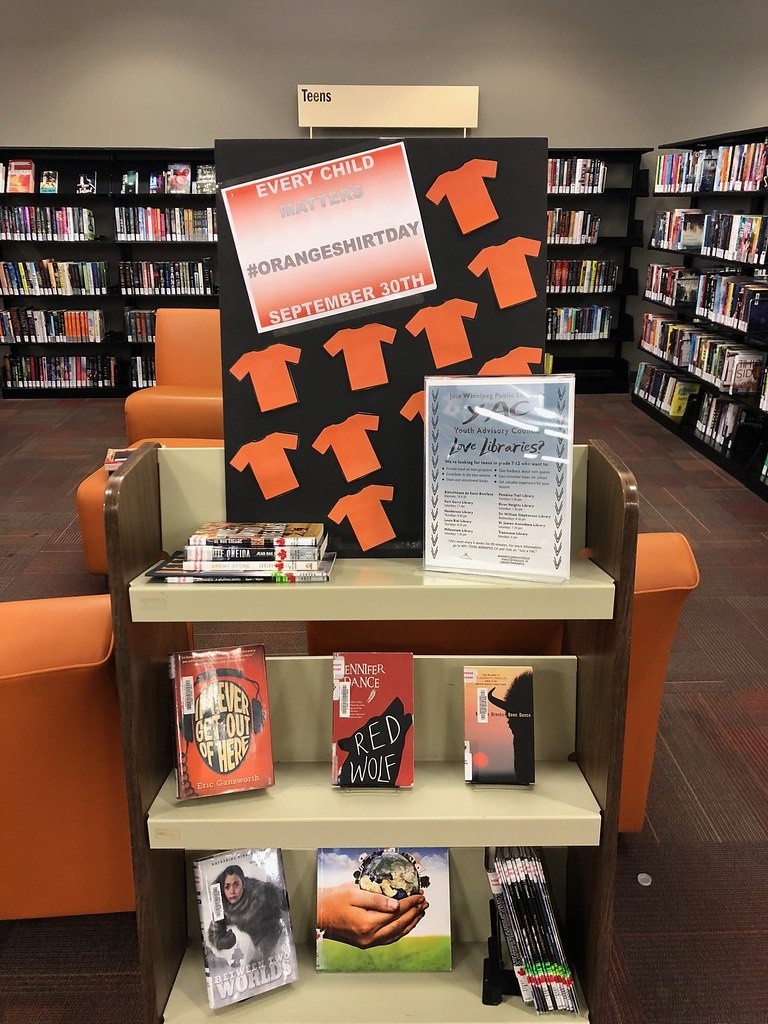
xmin=75 ymin=437 xmax=227 ymax=577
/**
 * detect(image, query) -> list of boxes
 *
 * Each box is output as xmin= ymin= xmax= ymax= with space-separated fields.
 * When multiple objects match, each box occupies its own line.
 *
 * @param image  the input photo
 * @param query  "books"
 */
xmin=633 ymin=137 xmax=767 ymax=487
xmin=332 ymin=652 xmax=413 ymax=789
xmin=0 ymin=158 xmax=218 ymax=388
xmin=169 ymin=643 xmax=275 ymax=800
xmin=545 ymin=156 xmax=620 ymax=341
xmin=145 ymin=521 xmax=337 ymax=583
xmin=194 ymin=847 xmax=299 ymax=1009
xmin=104 ymin=445 xmax=165 ymax=470
xmin=316 ymin=848 xmax=453 ymax=973
xmin=484 ymin=847 xmax=582 ymax=1019
xmin=464 ymin=664 xmax=536 ymax=786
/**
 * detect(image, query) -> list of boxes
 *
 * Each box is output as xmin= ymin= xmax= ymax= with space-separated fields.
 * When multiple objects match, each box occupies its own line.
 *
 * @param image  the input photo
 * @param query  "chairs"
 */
xmin=124 ymin=307 xmax=226 ymax=447
xmin=0 ymin=595 xmax=138 ymax=921
xmin=619 ymin=530 xmax=700 ymax=836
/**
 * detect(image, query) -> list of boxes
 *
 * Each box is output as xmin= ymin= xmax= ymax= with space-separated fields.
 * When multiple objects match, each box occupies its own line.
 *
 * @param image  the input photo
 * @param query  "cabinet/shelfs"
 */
xmin=543 ymin=146 xmax=655 ymax=396
xmin=630 ymin=125 xmax=768 ymax=505
xmin=1 ymin=148 xmax=215 ymax=401
xmin=102 ymin=439 xmax=641 ymax=1023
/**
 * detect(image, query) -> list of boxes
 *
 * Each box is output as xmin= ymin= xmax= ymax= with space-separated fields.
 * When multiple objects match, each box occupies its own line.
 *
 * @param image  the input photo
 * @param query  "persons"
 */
xmin=208 ymin=864 xmax=289 ymax=958
